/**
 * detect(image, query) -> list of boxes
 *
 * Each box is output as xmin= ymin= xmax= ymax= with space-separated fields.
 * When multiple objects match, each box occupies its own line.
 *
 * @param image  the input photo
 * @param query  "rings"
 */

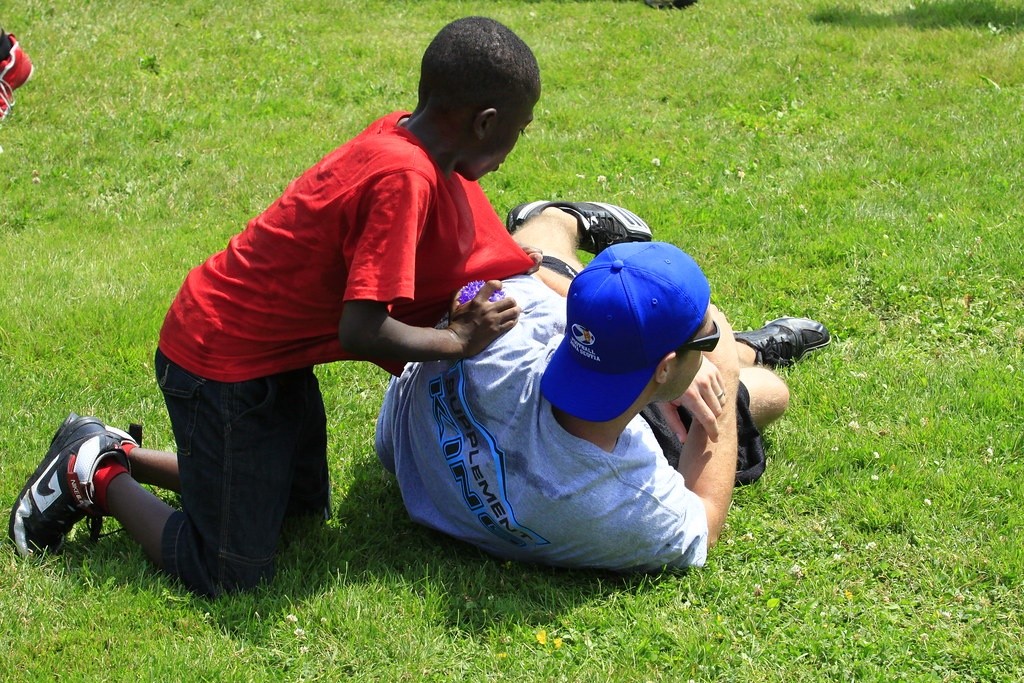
xmin=716 ymin=390 xmax=724 ymax=399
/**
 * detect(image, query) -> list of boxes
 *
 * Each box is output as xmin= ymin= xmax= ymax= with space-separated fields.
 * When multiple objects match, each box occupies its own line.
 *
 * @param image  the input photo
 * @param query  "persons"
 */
xmin=374 ymin=197 xmax=829 ymax=579
xmin=6 ymin=16 xmax=543 ymax=604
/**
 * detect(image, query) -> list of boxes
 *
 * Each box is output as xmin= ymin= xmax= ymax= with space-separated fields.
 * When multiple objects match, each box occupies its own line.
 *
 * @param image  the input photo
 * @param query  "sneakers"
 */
xmin=506 ymin=201 xmax=652 ymax=255
xmin=8 ymin=411 xmax=138 ymax=564
xmin=734 ymin=317 xmax=831 ymax=369
xmin=0 ymin=34 xmax=34 ymax=124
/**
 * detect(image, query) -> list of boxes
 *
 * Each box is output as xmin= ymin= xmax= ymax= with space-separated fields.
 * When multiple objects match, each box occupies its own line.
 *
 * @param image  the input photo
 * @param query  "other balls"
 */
xmin=456 ymin=280 xmax=506 ymax=305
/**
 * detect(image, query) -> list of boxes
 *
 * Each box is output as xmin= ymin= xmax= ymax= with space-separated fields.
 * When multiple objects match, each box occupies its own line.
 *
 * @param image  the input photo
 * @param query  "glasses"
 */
xmin=675 ymin=320 xmax=720 ymax=353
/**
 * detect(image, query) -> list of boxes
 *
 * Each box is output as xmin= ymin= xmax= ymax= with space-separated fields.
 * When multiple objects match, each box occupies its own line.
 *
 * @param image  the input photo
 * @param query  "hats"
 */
xmin=540 ymin=242 xmax=710 ymax=423
